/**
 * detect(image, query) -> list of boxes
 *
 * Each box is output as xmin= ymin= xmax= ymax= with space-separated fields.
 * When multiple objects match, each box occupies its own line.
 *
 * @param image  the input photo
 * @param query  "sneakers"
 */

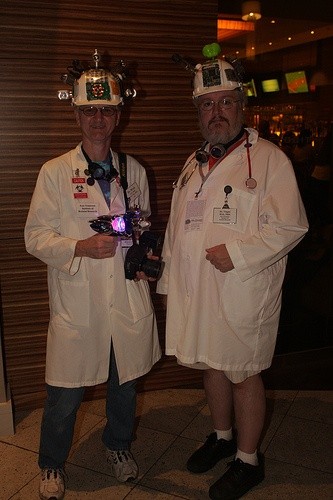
xmin=186 ymin=431 xmax=237 ymax=473
xmin=209 ymin=452 xmax=265 ymax=500
xmin=39 ymin=466 xmax=65 ymax=500
xmin=106 ymin=446 xmax=139 ymax=482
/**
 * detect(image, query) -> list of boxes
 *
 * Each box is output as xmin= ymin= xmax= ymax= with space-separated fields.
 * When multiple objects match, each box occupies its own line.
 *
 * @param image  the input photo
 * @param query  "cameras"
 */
xmin=124 ymin=244 xmax=165 ymax=281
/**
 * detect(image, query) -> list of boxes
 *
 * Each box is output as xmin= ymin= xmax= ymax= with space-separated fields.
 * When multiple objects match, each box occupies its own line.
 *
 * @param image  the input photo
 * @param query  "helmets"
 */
xmin=70 ymin=68 xmax=125 ymax=107
xmin=191 ymin=59 xmax=242 ymax=100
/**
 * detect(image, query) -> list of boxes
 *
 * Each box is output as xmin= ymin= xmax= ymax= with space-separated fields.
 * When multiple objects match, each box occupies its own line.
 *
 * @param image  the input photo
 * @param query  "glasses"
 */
xmin=198 ymin=96 xmax=242 ymax=111
xmin=78 ymin=107 xmax=119 ymax=116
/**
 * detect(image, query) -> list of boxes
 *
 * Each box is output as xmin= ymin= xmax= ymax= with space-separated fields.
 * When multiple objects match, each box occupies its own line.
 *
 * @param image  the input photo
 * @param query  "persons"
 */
xmin=23 ymin=69 xmax=162 ymax=500
xmin=156 ymin=59 xmax=308 ymax=500
